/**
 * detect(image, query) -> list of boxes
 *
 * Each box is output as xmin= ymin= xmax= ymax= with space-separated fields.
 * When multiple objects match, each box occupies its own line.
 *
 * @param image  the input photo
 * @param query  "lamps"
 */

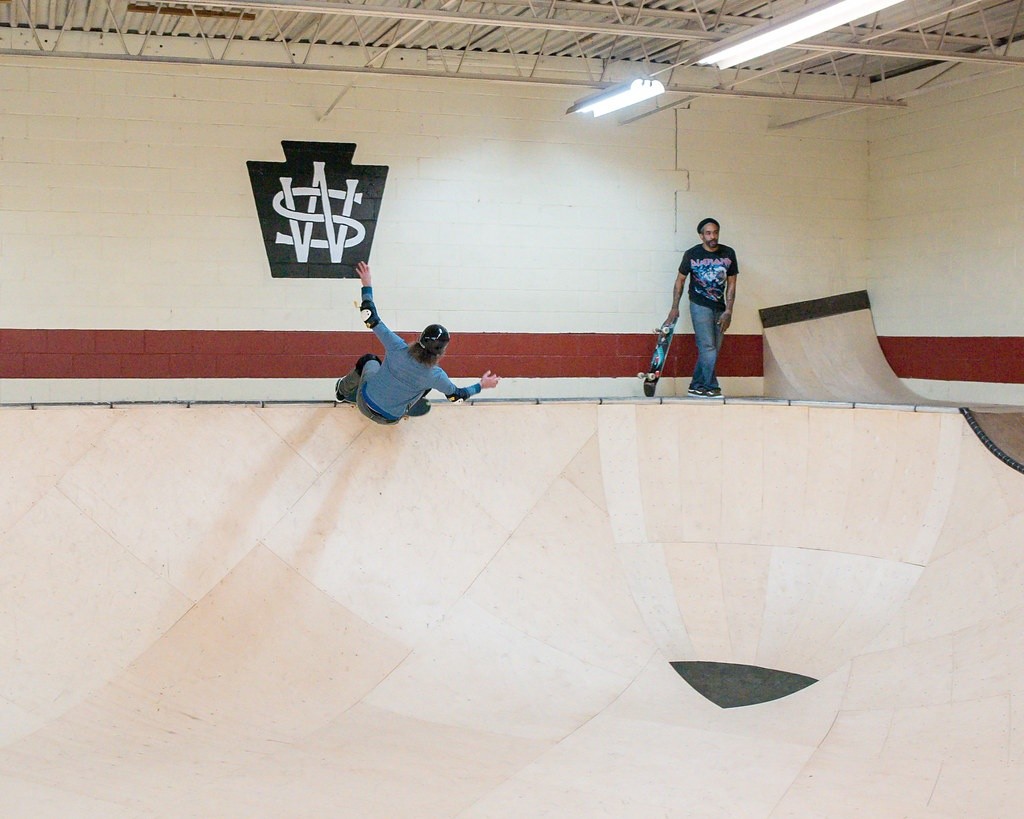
xmin=695 ymin=0 xmax=906 ymax=70
xmin=566 ymin=75 xmax=665 ymax=118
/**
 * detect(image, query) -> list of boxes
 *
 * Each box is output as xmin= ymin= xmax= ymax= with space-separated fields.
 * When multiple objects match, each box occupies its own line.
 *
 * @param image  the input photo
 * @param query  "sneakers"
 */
xmin=704 ymin=387 xmax=725 ymax=398
xmin=688 ymin=387 xmax=707 ymax=398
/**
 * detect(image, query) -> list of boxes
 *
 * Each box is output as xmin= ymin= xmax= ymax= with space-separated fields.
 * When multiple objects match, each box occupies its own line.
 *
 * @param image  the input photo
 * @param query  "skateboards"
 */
xmin=334 ymin=378 xmax=431 ymax=417
xmin=644 ymin=314 xmax=680 ymax=398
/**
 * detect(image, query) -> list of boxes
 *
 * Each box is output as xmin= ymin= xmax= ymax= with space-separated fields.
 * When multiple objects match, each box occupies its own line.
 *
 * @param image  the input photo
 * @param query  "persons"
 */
xmin=666 ymin=217 xmax=739 ymax=399
xmin=335 ymin=260 xmax=499 ymax=425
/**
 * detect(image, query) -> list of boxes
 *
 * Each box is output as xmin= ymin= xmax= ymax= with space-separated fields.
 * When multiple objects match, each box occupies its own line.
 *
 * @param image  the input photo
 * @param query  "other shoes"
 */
xmin=335 ymin=378 xmax=345 ymax=403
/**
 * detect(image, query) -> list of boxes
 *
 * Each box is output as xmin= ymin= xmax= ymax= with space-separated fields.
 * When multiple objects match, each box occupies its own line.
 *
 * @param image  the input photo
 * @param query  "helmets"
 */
xmin=418 ymin=324 xmax=451 ymax=356
